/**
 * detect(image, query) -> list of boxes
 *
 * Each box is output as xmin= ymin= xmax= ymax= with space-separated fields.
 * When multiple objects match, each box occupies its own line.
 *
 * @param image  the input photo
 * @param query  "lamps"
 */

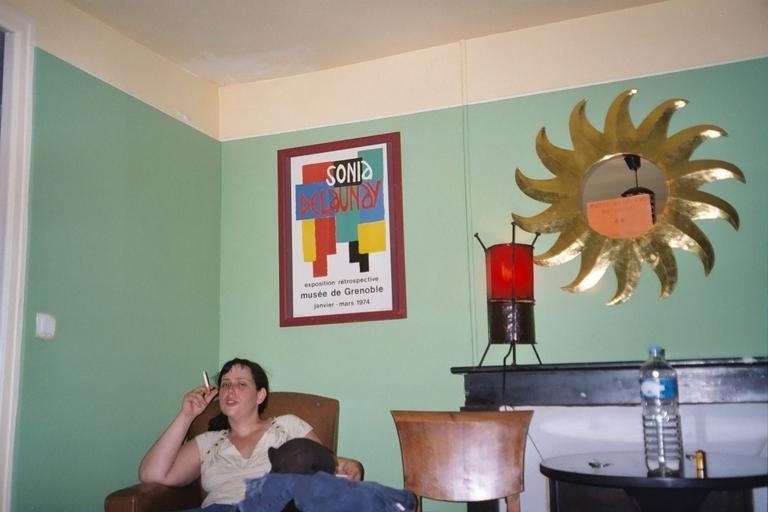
xmin=468 ymin=219 xmax=546 ymax=368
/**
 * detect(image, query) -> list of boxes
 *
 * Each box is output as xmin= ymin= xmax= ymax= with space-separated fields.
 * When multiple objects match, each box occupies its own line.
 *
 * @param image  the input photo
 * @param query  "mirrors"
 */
xmin=511 ymin=89 xmax=746 ymax=306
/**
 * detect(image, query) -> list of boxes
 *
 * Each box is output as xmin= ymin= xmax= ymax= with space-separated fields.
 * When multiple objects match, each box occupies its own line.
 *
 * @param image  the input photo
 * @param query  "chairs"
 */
xmin=105 ymin=391 xmax=344 ymax=512
xmin=387 ymin=409 xmax=538 ymax=512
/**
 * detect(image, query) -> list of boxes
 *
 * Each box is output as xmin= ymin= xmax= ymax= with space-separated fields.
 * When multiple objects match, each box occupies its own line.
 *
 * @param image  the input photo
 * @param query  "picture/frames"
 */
xmin=276 ymin=130 xmax=408 ymax=327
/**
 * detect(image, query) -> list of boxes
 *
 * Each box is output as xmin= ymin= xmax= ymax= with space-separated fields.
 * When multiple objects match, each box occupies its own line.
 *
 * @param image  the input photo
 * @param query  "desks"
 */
xmin=538 ymin=450 xmax=768 ymax=512
xmin=451 ymin=355 xmax=768 ymax=511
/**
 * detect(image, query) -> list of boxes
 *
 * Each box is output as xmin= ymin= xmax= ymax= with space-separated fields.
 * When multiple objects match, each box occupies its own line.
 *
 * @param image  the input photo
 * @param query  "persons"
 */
xmin=138 ymin=358 xmax=364 ymax=512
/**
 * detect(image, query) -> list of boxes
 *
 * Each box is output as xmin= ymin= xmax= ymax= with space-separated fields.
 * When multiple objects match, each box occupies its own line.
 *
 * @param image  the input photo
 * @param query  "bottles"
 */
xmin=639 ymin=344 xmax=682 ymax=475
xmin=694 ymin=451 xmax=706 ymax=471
xmin=646 ymin=473 xmax=683 ymax=482
xmin=697 ymin=470 xmax=707 ymax=478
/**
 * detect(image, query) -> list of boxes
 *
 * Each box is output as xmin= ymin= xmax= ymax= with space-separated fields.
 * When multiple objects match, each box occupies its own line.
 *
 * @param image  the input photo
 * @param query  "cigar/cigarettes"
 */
xmin=202 ymin=371 xmax=210 ymax=395
